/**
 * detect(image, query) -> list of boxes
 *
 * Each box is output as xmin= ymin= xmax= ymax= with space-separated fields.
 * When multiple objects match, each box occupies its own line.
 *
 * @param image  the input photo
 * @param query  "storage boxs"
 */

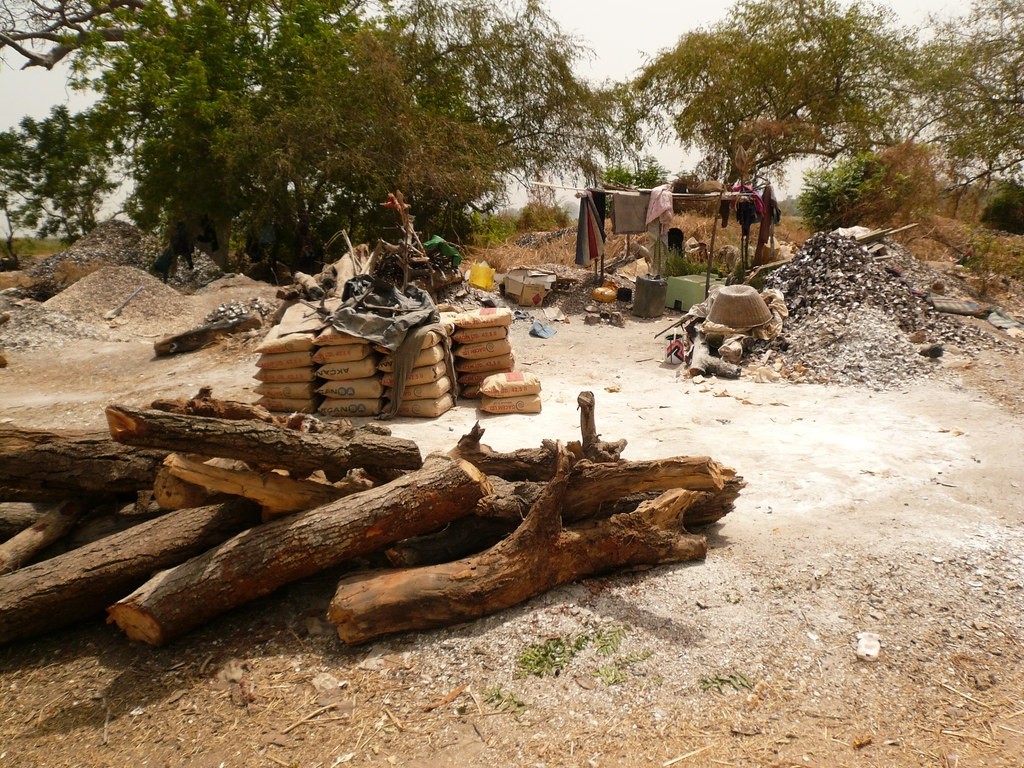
xmin=665 ymin=275 xmax=717 ymax=312
xmin=503 ymin=268 xmax=557 ymax=306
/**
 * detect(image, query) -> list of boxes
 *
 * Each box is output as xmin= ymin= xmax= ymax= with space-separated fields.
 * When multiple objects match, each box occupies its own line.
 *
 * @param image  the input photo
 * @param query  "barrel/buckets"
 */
xmin=632 ymin=273 xmax=668 ymax=317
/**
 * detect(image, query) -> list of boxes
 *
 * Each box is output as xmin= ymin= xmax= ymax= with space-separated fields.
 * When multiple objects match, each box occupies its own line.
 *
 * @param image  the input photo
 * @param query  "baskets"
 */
xmin=706 ymin=284 xmax=773 ymax=328
